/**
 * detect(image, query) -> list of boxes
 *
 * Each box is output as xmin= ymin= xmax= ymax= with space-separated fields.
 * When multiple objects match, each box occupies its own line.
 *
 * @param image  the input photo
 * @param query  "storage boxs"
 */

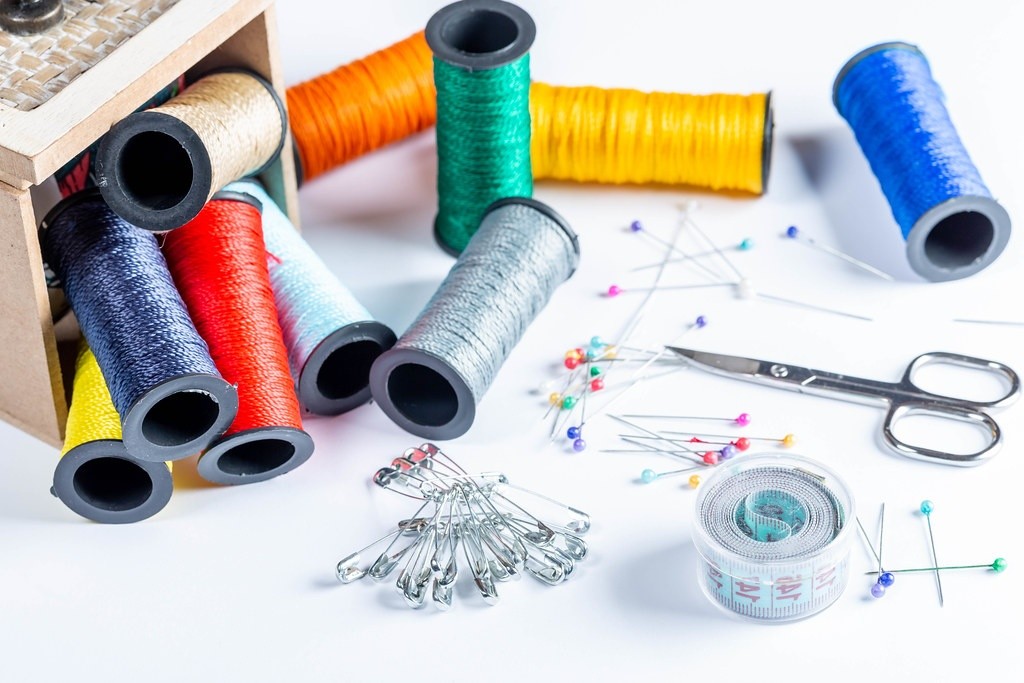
xmin=0 ymin=0 xmax=302 ymax=451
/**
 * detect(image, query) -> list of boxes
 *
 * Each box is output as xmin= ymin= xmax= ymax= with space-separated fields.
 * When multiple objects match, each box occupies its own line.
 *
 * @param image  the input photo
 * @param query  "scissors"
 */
xmin=664 ymin=337 xmax=1020 ymax=467
